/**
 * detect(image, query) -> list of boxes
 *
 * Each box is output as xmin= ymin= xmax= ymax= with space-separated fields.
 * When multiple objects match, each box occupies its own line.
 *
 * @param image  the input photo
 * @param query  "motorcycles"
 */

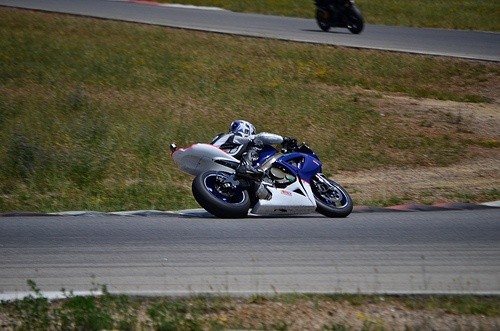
xmin=312 ymin=0 xmax=364 ymax=35
xmin=169 ymin=137 xmax=353 ymax=219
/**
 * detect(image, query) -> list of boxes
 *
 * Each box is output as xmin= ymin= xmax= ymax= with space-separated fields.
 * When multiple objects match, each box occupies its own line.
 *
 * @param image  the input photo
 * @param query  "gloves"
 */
xmin=282 ymin=136 xmax=297 ymax=147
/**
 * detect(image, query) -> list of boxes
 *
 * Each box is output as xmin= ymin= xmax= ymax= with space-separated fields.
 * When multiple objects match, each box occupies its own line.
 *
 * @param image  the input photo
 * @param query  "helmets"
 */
xmin=228 ymin=120 xmax=256 ymax=137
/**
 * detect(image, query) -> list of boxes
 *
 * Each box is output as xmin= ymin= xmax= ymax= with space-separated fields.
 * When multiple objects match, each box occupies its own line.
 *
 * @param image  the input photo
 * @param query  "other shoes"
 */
xmin=236 ymin=163 xmax=262 ymax=178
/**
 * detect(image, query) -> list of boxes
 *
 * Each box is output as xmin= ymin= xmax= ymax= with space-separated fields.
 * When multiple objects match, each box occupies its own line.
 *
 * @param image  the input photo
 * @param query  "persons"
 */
xmin=209 ymin=120 xmax=294 ymax=178
